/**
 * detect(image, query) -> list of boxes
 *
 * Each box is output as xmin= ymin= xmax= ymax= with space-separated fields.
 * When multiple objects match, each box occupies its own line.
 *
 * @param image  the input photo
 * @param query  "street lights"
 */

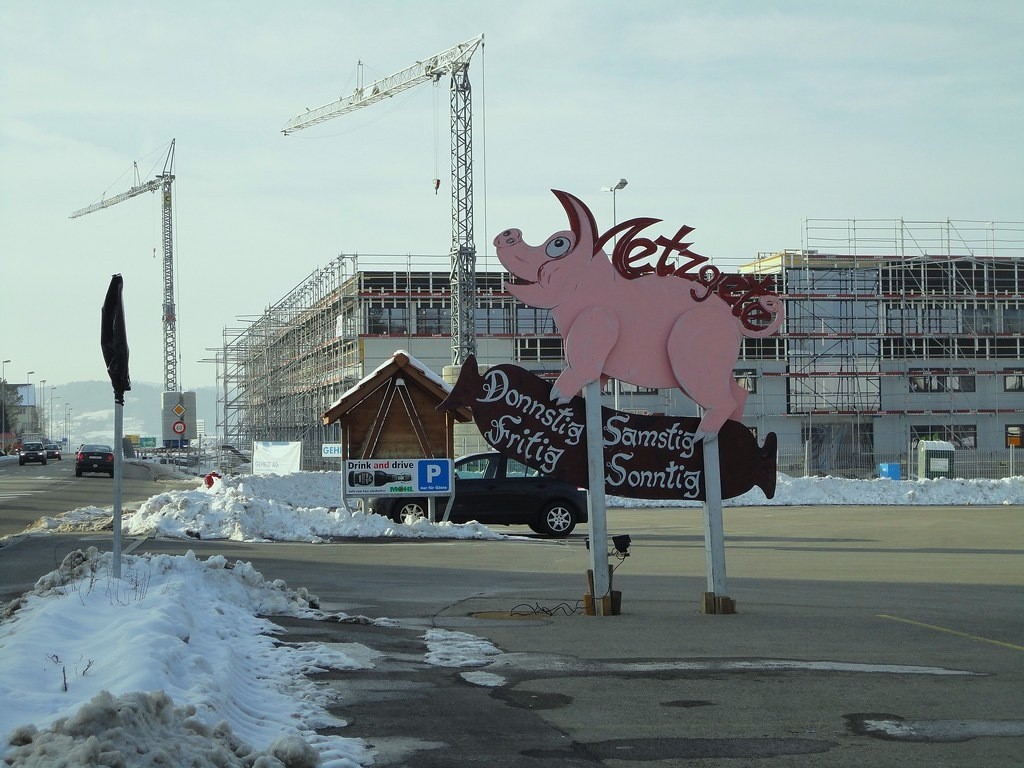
xmin=39 ymin=380 xmax=47 ymax=441
xmin=50 ymin=387 xmax=56 ymax=440
xmin=64 ymin=402 xmax=69 ymax=440
xmin=26 ymin=371 xmax=35 ymax=442
xmin=1 ymin=360 xmax=12 ymax=453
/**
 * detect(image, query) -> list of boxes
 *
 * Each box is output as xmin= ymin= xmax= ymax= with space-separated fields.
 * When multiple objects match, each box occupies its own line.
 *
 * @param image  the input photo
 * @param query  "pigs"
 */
xmin=492 ymin=187 xmax=784 ymax=442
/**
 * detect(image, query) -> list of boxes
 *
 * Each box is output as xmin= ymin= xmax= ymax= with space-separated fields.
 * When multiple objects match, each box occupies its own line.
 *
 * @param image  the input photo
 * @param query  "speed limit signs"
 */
xmin=173 ymin=421 xmax=186 ymax=434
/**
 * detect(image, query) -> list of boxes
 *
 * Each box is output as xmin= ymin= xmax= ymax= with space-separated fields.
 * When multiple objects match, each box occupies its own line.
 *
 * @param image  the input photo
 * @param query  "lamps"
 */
xmin=612 ymin=535 xmax=631 ymax=553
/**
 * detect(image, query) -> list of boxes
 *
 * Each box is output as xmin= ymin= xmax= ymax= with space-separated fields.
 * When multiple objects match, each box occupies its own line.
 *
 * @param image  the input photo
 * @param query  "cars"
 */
xmin=357 ymin=450 xmax=588 ymax=538
xmin=75 ymin=444 xmax=115 ymax=477
xmin=45 ymin=444 xmax=62 ymax=460
xmin=16 ymin=442 xmax=48 ymax=465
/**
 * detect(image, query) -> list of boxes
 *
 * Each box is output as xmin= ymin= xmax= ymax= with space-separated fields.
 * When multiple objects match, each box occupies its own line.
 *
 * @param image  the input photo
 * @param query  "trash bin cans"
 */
xmin=879 ymin=463 xmax=901 ymax=480
xmin=918 ymin=440 xmax=954 ymax=480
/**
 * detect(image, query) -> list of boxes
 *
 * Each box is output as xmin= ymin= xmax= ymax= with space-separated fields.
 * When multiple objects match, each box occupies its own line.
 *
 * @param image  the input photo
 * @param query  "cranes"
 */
xmin=280 ymin=30 xmax=490 ymax=365
xmin=68 ymin=138 xmax=177 ymax=391
